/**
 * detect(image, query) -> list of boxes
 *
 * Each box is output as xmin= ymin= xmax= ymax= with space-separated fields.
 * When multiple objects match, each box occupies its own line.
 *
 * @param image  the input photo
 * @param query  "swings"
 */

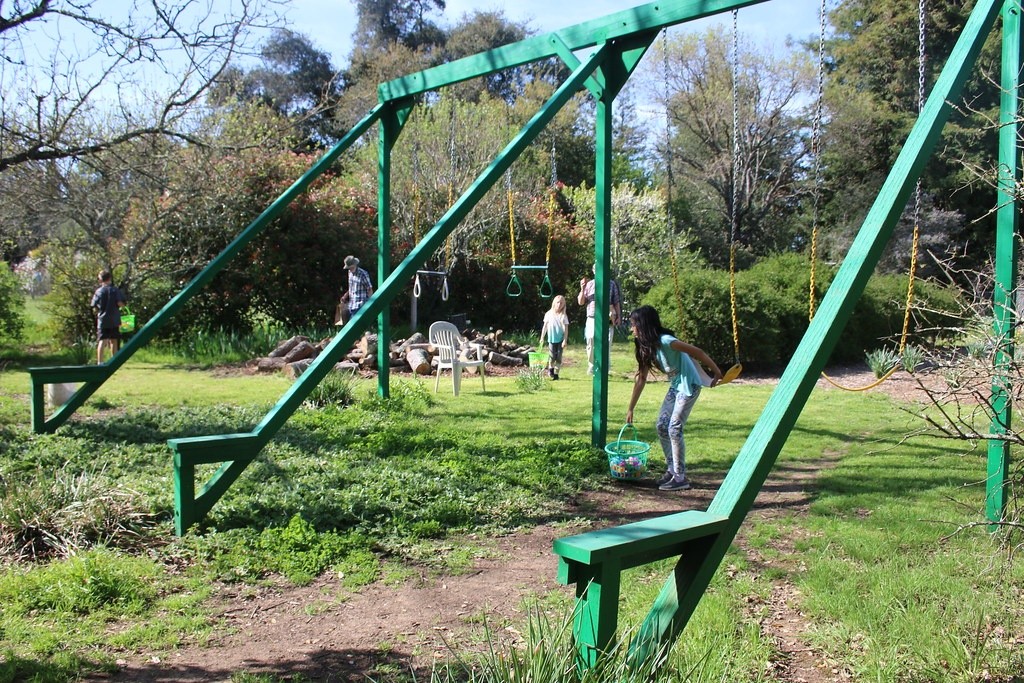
xmin=810 ymin=2 xmax=927 ymax=391
xmin=662 ymin=8 xmax=742 ymax=386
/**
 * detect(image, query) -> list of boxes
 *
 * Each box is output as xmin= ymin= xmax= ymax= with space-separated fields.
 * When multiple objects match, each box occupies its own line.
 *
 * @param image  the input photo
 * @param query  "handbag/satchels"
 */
xmin=334 ymin=301 xmax=345 ymax=326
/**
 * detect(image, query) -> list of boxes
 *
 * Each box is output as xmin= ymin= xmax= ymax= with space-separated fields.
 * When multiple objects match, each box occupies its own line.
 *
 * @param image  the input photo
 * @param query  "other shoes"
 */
xmin=553 ymin=374 xmax=558 ymax=380
xmin=548 ymin=367 xmax=554 ymax=377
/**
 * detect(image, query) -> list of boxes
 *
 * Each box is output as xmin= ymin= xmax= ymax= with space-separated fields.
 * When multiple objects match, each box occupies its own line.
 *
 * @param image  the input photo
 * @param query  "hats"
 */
xmin=343 ymin=256 xmax=359 ymax=269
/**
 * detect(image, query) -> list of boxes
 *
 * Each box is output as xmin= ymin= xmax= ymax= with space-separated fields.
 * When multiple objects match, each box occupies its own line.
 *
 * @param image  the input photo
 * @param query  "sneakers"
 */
xmin=659 ymin=475 xmax=690 ymax=491
xmin=656 ymin=471 xmax=676 ymax=486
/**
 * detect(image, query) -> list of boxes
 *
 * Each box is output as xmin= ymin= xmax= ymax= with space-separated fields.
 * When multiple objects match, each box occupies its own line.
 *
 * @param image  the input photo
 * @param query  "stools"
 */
xmin=429 ymin=320 xmax=485 ymax=395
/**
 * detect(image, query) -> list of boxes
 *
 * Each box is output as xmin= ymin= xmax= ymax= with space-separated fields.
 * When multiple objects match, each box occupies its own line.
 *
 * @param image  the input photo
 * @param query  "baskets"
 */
xmin=119 ymin=306 xmax=135 ymax=332
xmin=528 ymin=342 xmax=548 ymax=368
xmin=605 ymin=422 xmax=649 ymax=481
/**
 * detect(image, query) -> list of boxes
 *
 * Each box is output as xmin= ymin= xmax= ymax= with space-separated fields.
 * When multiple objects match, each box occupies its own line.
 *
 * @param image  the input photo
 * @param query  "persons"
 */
xmin=577 ymin=263 xmax=621 ymax=377
xmin=340 ymin=255 xmax=374 ymax=340
xmin=539 ymin=295 xmax=569 ymax=381
xmin=90 ymin=270 xmax=125 ymax=365
xmin=625 ymin=305 xmax=723 ymax=491
